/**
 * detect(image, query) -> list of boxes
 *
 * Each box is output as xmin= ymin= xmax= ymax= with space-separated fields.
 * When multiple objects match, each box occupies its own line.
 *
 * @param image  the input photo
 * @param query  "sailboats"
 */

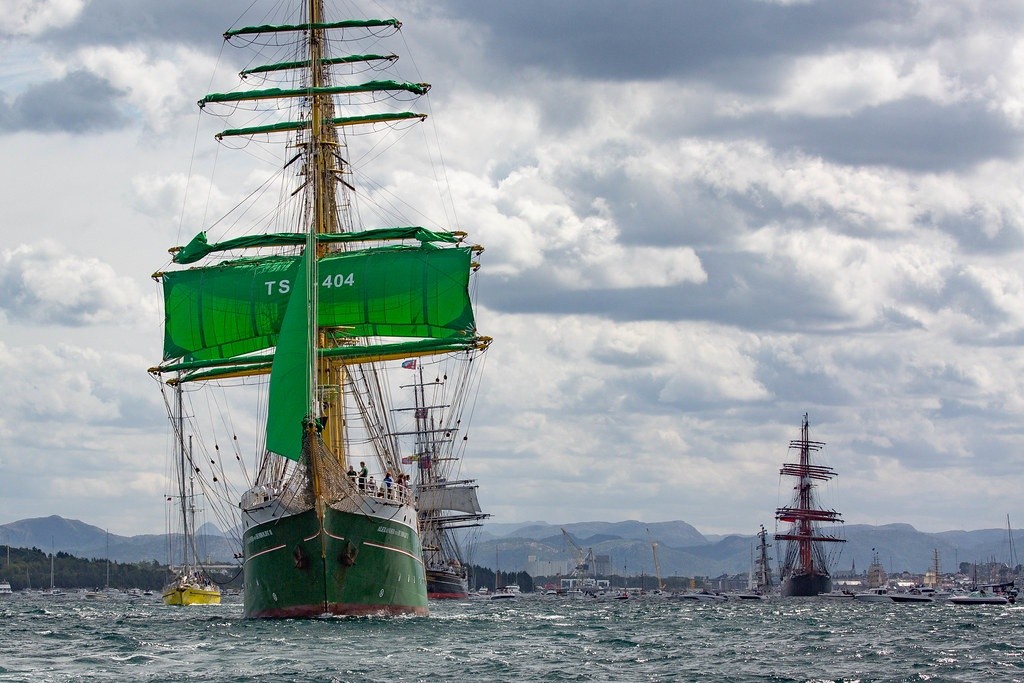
xmin=146 ymin=0 xmax=493 ymax=618
xmin=773 ymin=412 xmax=851 ymax=596
xmin=164 ymin=379 xmax=222 ymax=609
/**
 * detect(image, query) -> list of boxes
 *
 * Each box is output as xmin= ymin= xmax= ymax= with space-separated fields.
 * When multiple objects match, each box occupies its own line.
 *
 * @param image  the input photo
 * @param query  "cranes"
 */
xmin=559 ymin=528 xmax=587 ymax=570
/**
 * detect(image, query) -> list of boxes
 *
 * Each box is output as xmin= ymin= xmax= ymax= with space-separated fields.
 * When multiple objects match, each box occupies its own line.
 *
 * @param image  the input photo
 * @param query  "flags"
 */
xmin=402 ymin=359 xmax=416 ymax=369
xmin=403 ymin=409 xmax=433 ymax=469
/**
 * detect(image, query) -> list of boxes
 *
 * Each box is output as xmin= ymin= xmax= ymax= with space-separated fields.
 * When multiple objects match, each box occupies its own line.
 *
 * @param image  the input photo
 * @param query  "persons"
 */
xmin=347 ymin=462 xmax=410 ymax=505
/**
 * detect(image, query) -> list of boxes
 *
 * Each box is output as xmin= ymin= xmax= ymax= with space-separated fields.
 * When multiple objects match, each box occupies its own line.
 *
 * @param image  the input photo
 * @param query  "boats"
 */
xmin=20 ymin=529 xmax=156 ymax=600
xmin=472 ymin=525 xmax=780 ymax=601
xmin=821 ymin=515 xmax=1024 ymax=603
xmin=1 ymin=581 xmax=13 ymax=595
